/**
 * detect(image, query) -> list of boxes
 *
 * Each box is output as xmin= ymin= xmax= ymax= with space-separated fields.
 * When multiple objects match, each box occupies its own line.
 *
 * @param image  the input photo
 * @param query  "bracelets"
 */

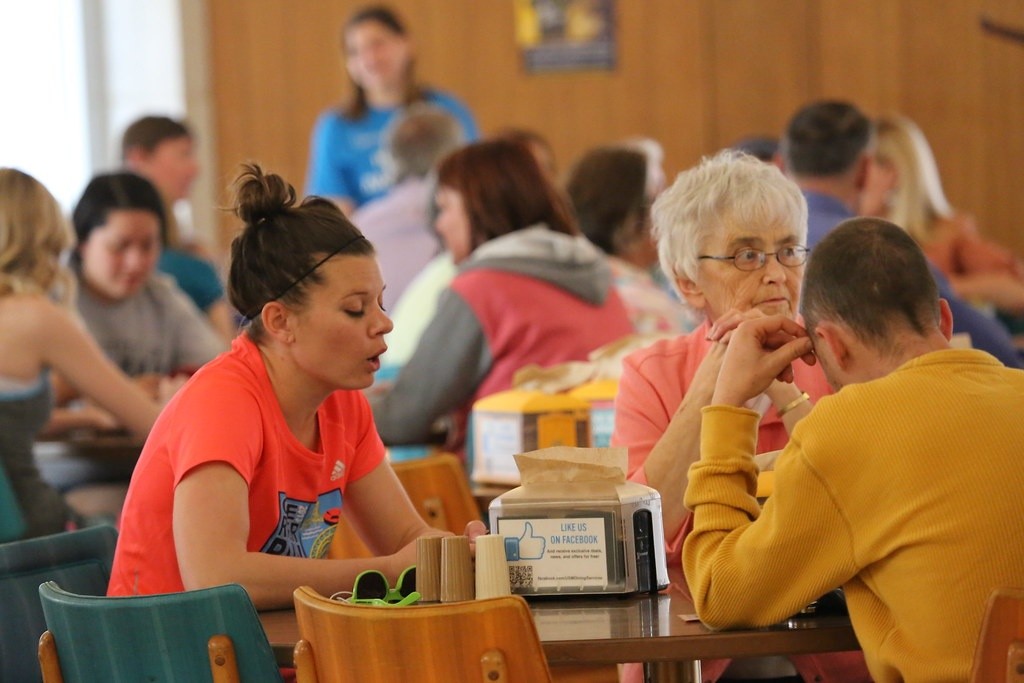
xmin=777 ymin=392 xmax=810 ymax=418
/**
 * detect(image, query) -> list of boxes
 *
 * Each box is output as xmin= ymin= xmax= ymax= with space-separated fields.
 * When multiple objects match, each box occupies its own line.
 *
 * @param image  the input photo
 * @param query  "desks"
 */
xmin=254 ymin=562 xmax=864 ymax=682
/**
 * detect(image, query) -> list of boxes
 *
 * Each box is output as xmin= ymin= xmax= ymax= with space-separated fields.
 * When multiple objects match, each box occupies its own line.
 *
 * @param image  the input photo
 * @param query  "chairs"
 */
xmin=0 ymin=524 xmax=120 ymax=683
xmin=293 ymin=585 xmax=554 ymax=683
xmin=37 ymin=581 xmax=285 ymax=683
xmin=324 ymin=454 xmax=481 ymax=565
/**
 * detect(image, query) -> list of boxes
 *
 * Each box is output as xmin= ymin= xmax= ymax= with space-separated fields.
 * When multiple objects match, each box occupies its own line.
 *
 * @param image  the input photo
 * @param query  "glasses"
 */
xmin=697 ymin=240 xmax=811 ymax=272
xmin=348 ymin=564 xmax=420 ymax=608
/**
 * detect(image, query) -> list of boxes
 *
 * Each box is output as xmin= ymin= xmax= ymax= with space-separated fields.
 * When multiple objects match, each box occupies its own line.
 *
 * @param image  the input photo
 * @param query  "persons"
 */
xmin=0 ymin=164 xmax=167 ymax=538
xmin=781 ymin=98 xmax=1024 ymax=364
xmin=104 ymin=166 xmax=486 ymax=609
xmin=117 ymin=113 xmax=237 ymax=339
xmin=569 ymin=140 xmax=698 ymax=338
xmin=682 ymin=215 xmax=1024 ymax=683
xmin=614 ymin=146 xmax=838 ymax=568
xmin=303 ymin=9 xmax=483 ymax=328
xmin=369 ymin=130 xmax=635 ymax=464
xmin=33 ymin=170 xmax=234 ymax=494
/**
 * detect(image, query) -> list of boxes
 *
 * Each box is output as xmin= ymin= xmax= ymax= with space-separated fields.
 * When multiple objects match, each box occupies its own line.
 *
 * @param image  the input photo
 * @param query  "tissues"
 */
xmin=489 ymin=445 xmax=671 ymax=595
